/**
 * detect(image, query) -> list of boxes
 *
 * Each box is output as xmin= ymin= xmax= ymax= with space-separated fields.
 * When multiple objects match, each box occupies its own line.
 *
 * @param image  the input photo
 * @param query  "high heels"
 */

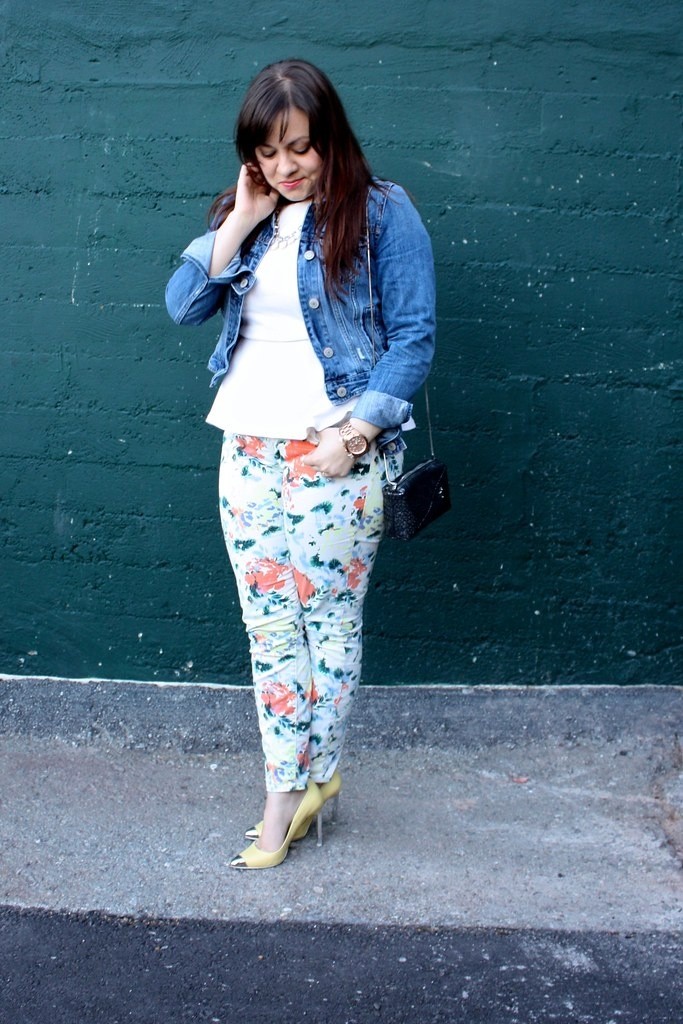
xmin=229 ymin=774 xmax=324 ymax=869
xmin=246 ymin=769 xmax=342 ymax=846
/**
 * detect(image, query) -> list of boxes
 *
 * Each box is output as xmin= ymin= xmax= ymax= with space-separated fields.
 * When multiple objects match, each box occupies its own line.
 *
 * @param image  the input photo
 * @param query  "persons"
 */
xmin=166 ymin=59 xmax=436 ymax=868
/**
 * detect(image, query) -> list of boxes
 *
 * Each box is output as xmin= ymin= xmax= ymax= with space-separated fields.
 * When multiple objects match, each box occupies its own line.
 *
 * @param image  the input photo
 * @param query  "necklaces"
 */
xmin=271 ymin=209 xmax=302 ymax=249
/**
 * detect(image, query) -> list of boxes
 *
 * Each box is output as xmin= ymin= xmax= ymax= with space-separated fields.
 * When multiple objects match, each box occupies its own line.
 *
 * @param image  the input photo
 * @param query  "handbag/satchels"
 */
xmin=381 ymin=456 xmax=451 ymax=541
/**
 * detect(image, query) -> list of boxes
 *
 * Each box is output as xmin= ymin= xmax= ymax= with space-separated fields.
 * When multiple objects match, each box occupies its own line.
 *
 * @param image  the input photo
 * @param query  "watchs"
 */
xmin=340 ymin=421 xmax=370 ymax=458
